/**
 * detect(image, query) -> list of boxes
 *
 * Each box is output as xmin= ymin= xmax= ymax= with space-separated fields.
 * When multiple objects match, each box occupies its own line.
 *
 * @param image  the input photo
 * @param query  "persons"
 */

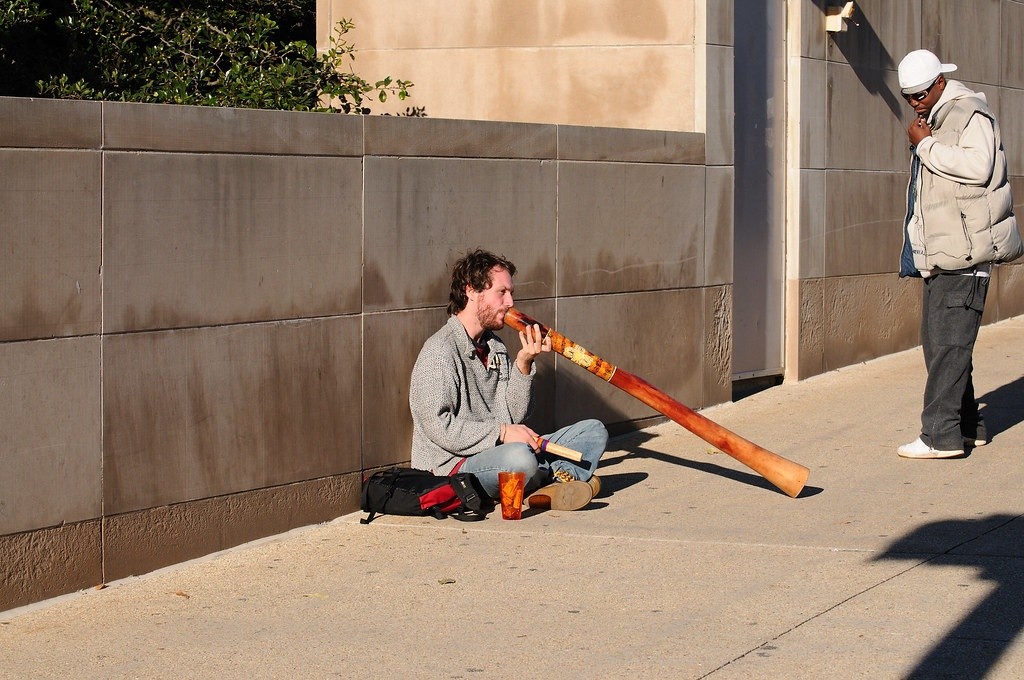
xmin=895 ymin=50 xmax=1024 ymax=459
xmin=408 ymin=249 xmax=609 ymax=511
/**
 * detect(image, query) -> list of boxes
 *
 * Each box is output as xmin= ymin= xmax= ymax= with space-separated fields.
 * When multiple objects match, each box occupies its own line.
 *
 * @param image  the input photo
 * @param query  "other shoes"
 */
xmin=961 ymin=435 xmax=987 ymax=446
xmin=522 ymin=481 xmax=593 ymax=511
xmin=897 ymin=437 xmax=965 ymax=459
xmin=550 ymin=470 xmax=601 ymax=498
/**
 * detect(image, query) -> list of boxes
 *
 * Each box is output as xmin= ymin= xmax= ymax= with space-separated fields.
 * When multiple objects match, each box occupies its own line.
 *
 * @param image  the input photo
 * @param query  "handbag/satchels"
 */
xmin=359 ymin=466 xmax=495 ymax=525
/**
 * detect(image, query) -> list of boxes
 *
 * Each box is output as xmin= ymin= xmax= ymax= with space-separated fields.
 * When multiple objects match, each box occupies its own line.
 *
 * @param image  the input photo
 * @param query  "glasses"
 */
xmin=900 ymin=75 xmax=941 ymax=103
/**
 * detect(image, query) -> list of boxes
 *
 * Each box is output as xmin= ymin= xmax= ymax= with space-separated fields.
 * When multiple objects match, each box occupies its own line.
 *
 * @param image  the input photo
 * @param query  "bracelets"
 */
xmin=502 ymin=423 xmax=507 ymax=443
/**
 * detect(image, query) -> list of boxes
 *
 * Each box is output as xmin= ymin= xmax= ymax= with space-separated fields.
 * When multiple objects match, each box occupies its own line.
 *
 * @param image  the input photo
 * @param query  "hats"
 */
xmin=898 ymin=49 xmax=958 ymax=94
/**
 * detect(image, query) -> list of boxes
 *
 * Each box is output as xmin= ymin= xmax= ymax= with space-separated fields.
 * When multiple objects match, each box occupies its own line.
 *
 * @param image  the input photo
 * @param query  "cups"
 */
xmin=499 ymin=472 xmax=526 ymax=520
